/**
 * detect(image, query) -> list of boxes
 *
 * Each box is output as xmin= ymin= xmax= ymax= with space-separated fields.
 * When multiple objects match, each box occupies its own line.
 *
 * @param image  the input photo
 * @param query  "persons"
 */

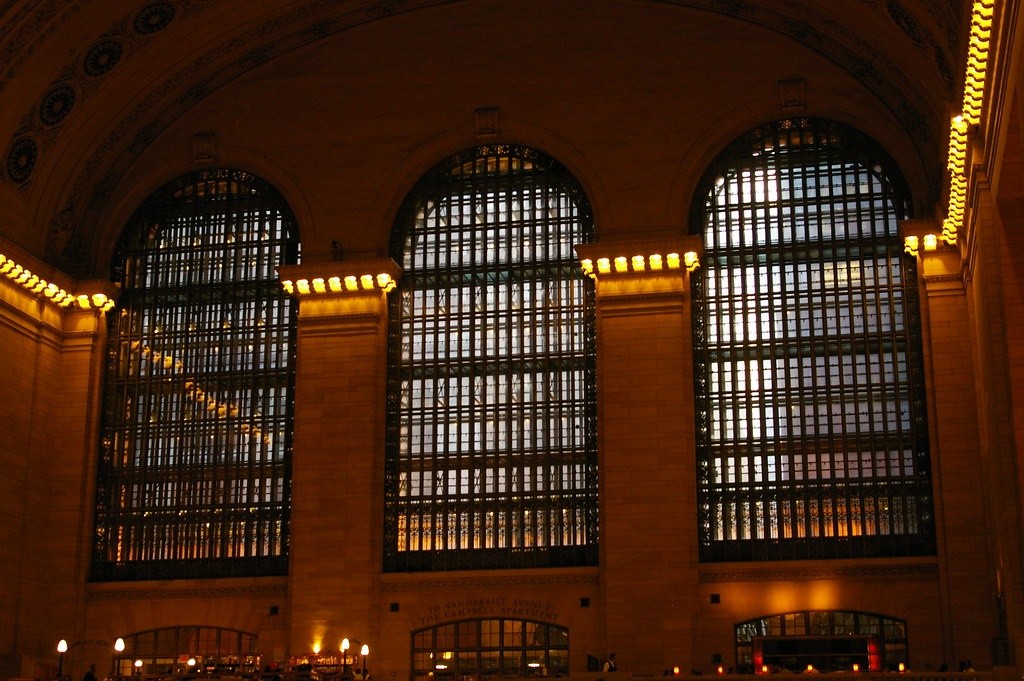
xmin=82 ymin=664 xmax=98 ymax=681
xmin=55 ymin=674 xmax=62 ymax=681
xmin=63 ymin=674 xmax=71 ymax=681
xmin=264 ymin=659 xmax=373 ymax=680
xmin=134 ymin=672 xmax=146 ymax=681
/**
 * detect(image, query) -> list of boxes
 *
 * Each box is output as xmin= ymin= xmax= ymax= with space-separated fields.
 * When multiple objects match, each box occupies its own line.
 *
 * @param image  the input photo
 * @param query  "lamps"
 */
xmin=115 ymin=637 xmax=124 ymax=679
xmin=807 ymin=665 xmax=813 ymax=673
xmin=187 ymin=657 xmax=196 ymax=674
xmin=762 ymin=666 xmax=767 ymax=676
xmin=339 ymin=646 xmax=346 ymax=656
xmin=675 ymin=666 xmax=679 ymax=675
xmin=852 ymin=663 xmax=860 ymax=672
xmin=311 ymin=644 xmax=324 ymax=656
xmin=899 ymin=661 xmax=904 ymax=673
xmin=717 ymin=665 xmax=722 ymax=675
xmin=443 ymin=651 xmax=453 ymax=660
xmin=55 ymin=638 xmax=69 ymax=681
xmin=133 ymin=660 xmax=144 ymax=676
xmin=342 ymin=637 xmax=350 ymax=681
xmin=361 ymin=643 xmax=370 ymax=681
